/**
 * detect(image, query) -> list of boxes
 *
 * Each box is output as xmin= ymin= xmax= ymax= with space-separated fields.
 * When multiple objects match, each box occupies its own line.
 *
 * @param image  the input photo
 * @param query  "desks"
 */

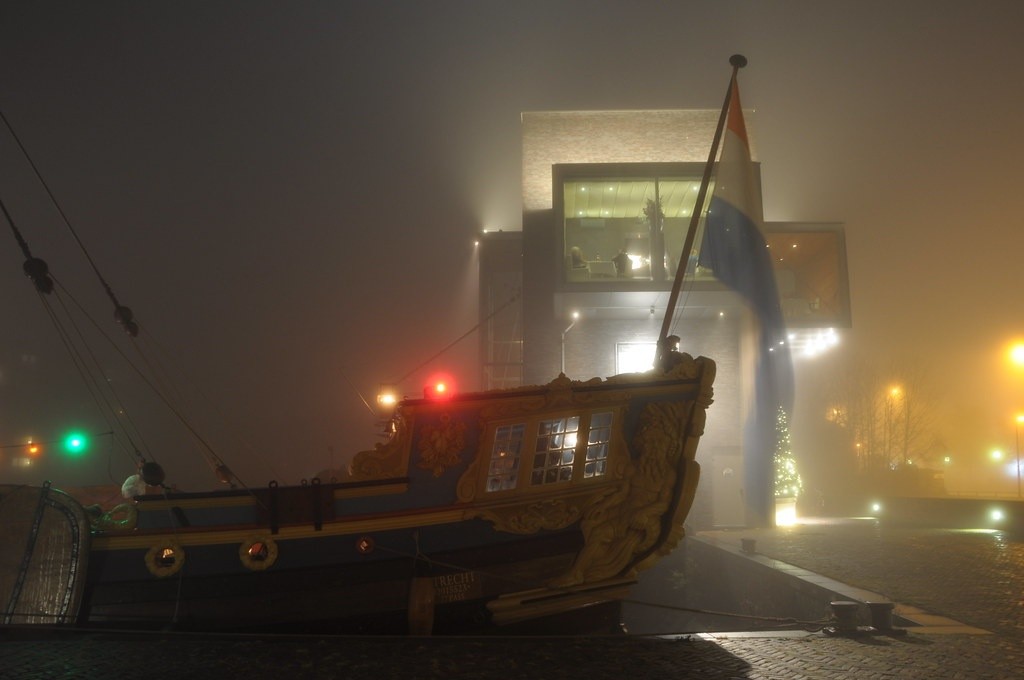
xmin=583 ymin=260 xmax=618 ymax=279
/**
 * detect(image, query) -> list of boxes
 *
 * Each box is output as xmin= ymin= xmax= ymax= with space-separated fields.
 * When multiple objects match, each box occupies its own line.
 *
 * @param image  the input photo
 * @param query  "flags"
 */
xmin=697 ymin=76 xmax=796 ymax=418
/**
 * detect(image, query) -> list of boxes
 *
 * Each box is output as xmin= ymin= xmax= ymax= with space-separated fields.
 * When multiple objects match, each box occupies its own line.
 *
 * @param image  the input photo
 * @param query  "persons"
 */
xmin=612 ymin=249 xmax=631 ymax=274
xmin=570 ymin=247 xmax=588 ymax=269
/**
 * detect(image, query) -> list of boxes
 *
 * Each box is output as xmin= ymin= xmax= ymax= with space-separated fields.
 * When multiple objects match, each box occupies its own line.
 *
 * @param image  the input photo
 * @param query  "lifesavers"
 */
xmin=238 ymin=533 xmax=279 ymax=573
xmin=144 ymin=539 xmax=187 ymax=578
xmin=99 ymin=503 xmax=140 ymax=531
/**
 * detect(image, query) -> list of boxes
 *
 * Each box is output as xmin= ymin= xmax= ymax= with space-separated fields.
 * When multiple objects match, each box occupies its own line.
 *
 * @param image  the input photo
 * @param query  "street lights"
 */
xmin=1015 ymin=413 xmax=1024 ymax=497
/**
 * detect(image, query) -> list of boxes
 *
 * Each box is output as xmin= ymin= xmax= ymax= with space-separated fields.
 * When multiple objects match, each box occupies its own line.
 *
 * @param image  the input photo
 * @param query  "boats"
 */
xmin=1 ymin=50 xmax=751 ymax=646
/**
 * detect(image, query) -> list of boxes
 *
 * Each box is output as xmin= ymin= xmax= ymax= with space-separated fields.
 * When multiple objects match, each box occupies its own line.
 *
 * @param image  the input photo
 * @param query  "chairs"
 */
xmin=565 ymin=255 xmax=589 ymax=279
